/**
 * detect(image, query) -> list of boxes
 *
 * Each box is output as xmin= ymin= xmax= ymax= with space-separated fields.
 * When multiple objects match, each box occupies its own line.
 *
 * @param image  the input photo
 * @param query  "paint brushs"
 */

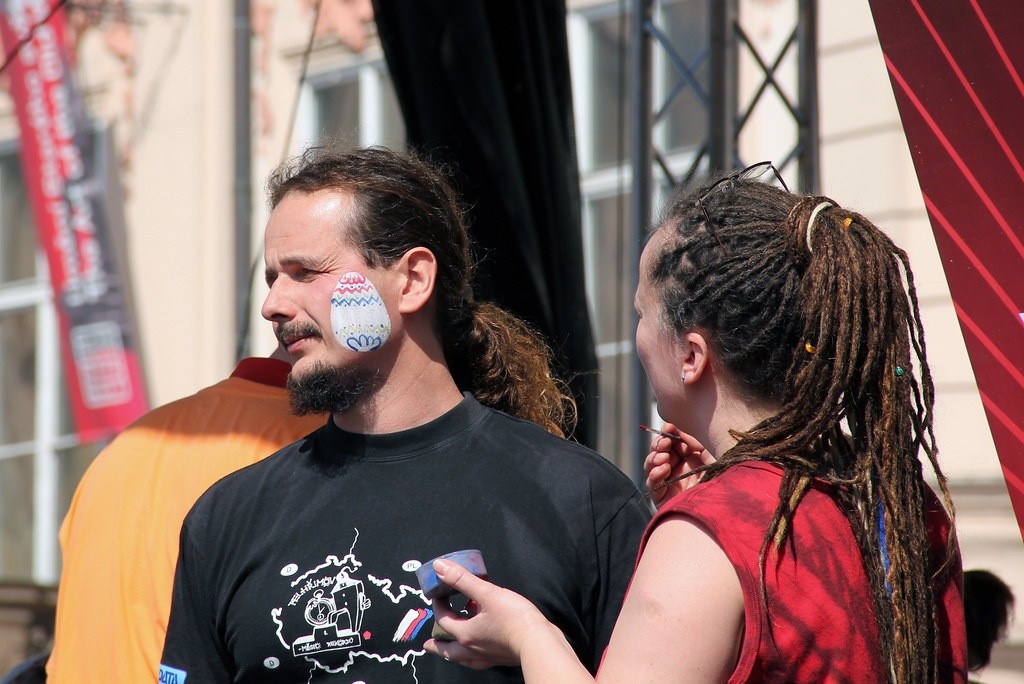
xmin=639 ymin=423 xmax=680 ymax=442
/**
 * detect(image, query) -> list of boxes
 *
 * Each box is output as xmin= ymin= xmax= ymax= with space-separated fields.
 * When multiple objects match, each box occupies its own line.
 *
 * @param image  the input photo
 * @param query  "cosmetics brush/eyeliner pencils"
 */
xmin=640 ymin=426 xmax=681 ymax=441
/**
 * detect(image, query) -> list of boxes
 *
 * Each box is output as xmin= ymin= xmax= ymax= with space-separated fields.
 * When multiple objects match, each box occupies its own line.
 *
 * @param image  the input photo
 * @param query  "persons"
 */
xmin=45 ymin=344 xmax=331 ymax=684
xmin=963 ymin=571 xmax=1012 ymax=669
xmin=156 ymin=149 xmax=653 ymax=684
xmin=422 ymin=178 xmax=967 ymax=684
